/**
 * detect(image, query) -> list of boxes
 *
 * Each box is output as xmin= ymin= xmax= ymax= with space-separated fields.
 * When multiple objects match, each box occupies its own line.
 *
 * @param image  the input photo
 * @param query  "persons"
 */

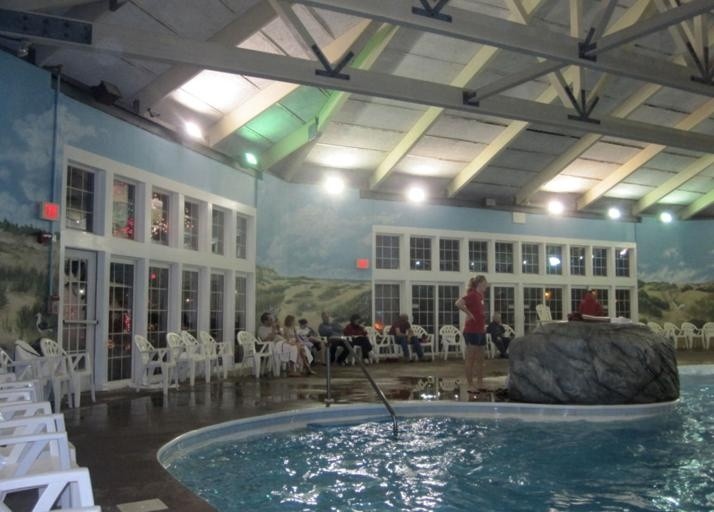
xmin=453 ymin=274 xmax=490 ymax=395
xmin=319 ymin=312 xmax=353 ymax=367
xmin=578 ymin=289 xmax=604 ymax=317
xmin=343 ymin=314 xmax=375 ymax=366
xmin=297 ymin=317 xmax=328 ymax=366
xmin=258 ymin=311 xmax=318 ymax=377
xmin=284 ymin=315 xmax=314 ymax=369
xmin=389 ymin=314 xmax=430 ymax=362
xmin=487 ymin=312 xmax=513 ymax=360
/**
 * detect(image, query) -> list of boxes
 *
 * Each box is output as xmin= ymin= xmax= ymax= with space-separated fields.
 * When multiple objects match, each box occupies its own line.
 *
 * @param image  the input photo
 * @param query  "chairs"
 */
xmin=0 ymin=337 xmax=101 ymax=511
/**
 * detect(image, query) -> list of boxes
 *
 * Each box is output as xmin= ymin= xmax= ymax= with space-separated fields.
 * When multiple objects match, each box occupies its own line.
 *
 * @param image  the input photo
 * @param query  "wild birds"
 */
xmin=34 ymin=313 xmax=52 ymax=334
